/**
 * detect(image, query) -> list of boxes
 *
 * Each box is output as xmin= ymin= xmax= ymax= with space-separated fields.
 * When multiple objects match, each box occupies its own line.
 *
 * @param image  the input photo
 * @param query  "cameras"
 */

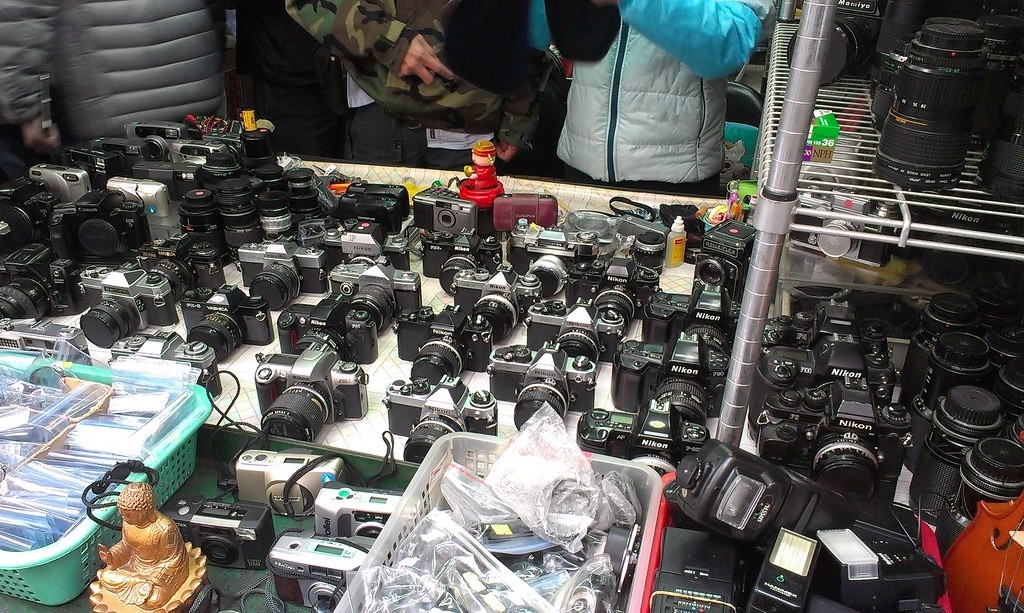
xmin=0 ymin=0 xmax=946 ymax=613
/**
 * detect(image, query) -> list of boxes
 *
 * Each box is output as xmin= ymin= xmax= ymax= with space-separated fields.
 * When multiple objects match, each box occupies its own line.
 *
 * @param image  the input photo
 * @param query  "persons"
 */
xmin=0 ymin=1 xmax=228 ymax=168
xmin=238 ymin=1 xmax=761 ymax=199
xmin=96 ymin=479 xmax=189 ymax=611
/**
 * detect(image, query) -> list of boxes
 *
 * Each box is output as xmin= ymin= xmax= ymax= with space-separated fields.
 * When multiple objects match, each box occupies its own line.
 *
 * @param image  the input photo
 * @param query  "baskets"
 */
xmin=640 ymin=470 xmax=950 ymax=612
xmin=1 ymin=350 xmax=214 ymax=607
xmin=332 ymin=431 xmax=663 ymax=612
